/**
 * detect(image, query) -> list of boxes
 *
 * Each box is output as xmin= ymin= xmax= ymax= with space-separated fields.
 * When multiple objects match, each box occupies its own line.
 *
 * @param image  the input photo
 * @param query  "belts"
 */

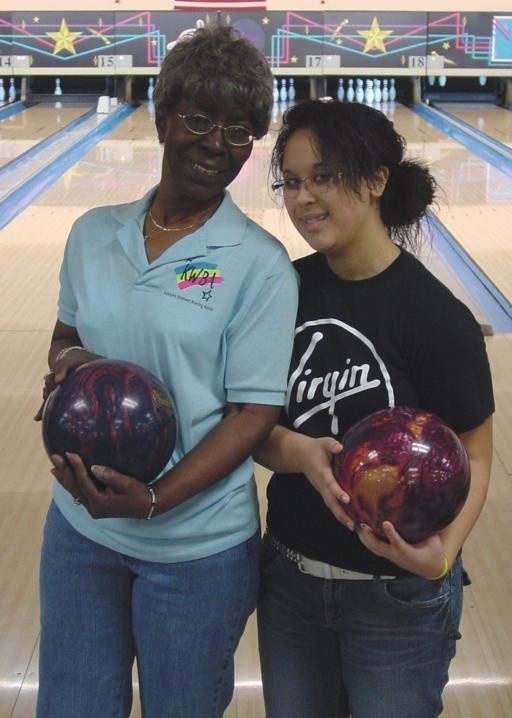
xmin=263 ymin=533 xmax=399 ymax=591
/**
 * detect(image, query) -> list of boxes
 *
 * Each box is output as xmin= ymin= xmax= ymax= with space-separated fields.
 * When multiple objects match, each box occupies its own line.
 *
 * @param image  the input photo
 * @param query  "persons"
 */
xmin=252 ymin=96 xmax=495 ymax=718
xmin=36 ymin=23 xmax=301 ymax=717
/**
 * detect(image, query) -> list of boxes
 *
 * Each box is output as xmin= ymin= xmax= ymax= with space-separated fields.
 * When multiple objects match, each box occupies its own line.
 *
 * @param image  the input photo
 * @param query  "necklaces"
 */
xmin=148 ymin=209 xmax=210 ymax=232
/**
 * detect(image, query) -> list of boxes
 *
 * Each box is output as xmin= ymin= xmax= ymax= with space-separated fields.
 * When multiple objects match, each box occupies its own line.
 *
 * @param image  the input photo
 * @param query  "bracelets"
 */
xmin=146 ymin=486 xmax=156 ymax=521
xmin=425 ymin=556 xmax=449 ymax=581
xmin=56 ymin=345 xmax=85 ymax=361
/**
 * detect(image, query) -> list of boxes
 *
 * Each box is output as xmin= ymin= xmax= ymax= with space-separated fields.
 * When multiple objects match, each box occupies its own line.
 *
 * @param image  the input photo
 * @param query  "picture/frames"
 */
xmin=491 ymin=15 xmax=512 ymax=63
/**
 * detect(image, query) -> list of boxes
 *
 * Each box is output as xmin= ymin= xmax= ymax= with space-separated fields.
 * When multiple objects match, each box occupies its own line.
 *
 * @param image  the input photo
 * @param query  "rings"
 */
xmin=73 ymin=495 xmax=81 ymax=506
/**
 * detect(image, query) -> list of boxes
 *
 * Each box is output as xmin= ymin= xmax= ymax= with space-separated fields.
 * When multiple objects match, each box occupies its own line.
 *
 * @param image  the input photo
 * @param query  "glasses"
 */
xmin=270 ymin=173 xmax=341 ymax=199
xmin=168 ymin=104 xmax=261 ymax=148
